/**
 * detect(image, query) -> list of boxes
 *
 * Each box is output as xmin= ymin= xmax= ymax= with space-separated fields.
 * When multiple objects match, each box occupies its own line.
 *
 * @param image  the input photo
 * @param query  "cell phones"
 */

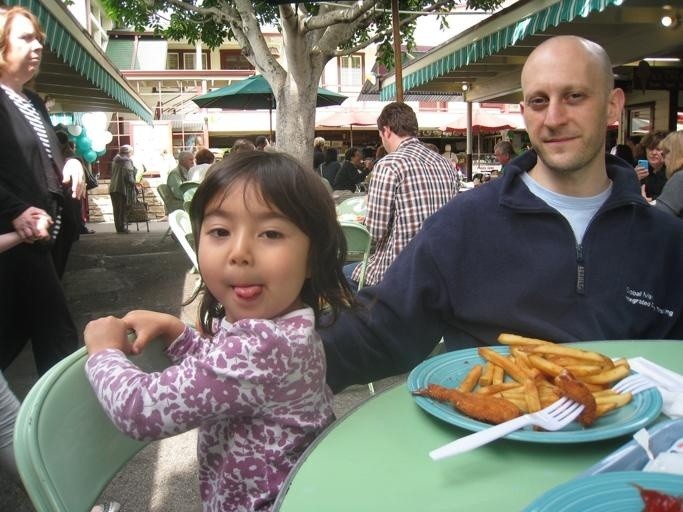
xmin=637 ymin=160 xmax=649 ymax=169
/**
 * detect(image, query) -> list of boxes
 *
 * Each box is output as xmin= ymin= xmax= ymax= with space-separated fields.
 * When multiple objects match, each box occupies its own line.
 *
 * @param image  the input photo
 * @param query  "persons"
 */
xmin=167 ymin=136 xmax=273 ymax=199
xmin=83 ymin=150 xmax=366 ymax=512
xmin=1 ymin=6 xmax=100 ymax=495
xmin=109 ymin=144 xmax=139 ymax=235
xmin=313 ymin=36 xmax=683 ymax=393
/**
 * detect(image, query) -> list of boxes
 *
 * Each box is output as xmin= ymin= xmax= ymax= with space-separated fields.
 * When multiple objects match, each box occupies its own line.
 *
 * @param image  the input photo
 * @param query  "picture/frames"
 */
xmin=627 ymin=101 xmax=655 ymax=141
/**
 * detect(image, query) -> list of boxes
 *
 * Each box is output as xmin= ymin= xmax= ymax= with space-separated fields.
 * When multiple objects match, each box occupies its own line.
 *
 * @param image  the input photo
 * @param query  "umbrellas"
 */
xmin=191 ymin=74 xmax=349 ymax=145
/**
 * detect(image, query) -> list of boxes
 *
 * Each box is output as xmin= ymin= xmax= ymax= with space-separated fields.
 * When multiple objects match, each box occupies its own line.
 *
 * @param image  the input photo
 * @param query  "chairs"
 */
xmin=168 ymin=209 xmax=198 ymax=269
xmin=12 ymin=323 xmax=174 ymax=512
xmin=337 ymin=222 xmax=375 ymax=397
xmin=178 ymin=181 xmax=201 ymax=214
xmin=157 ymin=184 xmax=179 ymax=243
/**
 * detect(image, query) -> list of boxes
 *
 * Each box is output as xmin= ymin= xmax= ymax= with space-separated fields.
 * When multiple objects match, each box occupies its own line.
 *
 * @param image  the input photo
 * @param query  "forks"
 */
xmin=611 ymin=372 xmax=658 ymax=396
xmin=428 ymin=394 xmax=586 ymax=462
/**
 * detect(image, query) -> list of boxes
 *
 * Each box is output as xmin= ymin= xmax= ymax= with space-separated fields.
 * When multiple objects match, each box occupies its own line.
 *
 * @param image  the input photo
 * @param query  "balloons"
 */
xmin=67 ymin=112 xmax=115 ymax=164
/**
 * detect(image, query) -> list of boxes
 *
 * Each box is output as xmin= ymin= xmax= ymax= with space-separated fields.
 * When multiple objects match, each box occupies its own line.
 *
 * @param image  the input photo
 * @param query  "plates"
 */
xmin=519 ymin=470 xmax=683 ymax=512
xmin=407 ymin=345 xmax=663 ymax=445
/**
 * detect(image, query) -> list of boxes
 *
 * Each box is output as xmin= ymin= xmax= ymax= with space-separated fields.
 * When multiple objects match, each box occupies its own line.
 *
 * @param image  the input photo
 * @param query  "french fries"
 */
xmin=460 ymin=333 xmax=632 ymax=434
